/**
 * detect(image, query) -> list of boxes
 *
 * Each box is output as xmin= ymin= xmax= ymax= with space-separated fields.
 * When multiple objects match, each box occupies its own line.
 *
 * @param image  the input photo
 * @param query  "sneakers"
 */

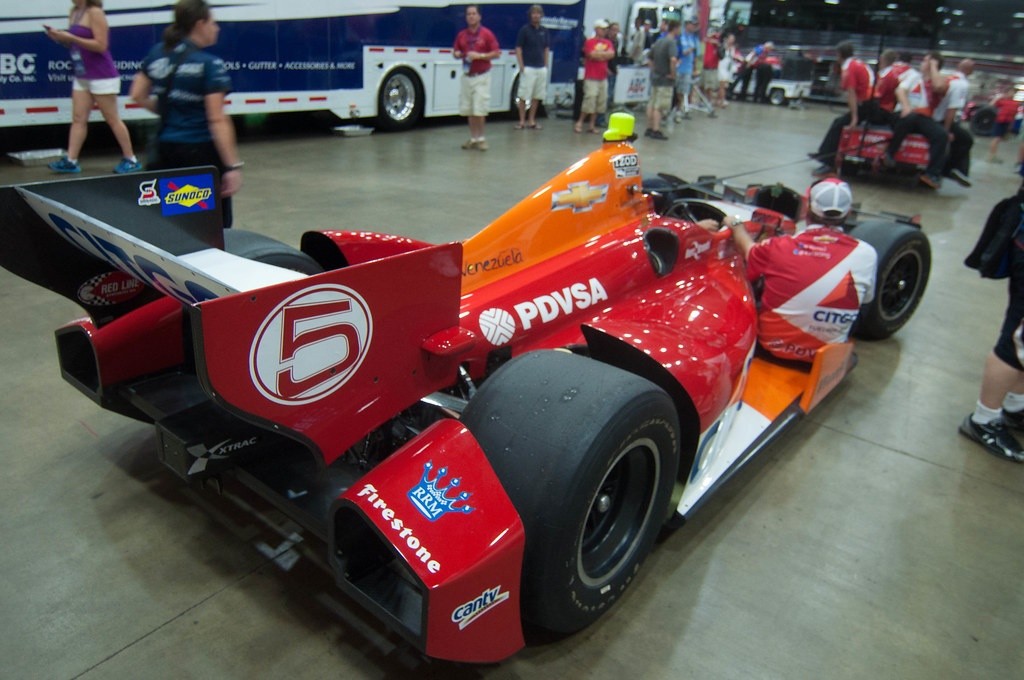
xmin=919 ymin=172 xmax=943 ymax=190
xmin=47 ymin=154 xmax=82 ymax=173
xmin=957 ymin=412 xmax=1024 ymax=464
xmin=948 ymin=167 xmax=972 ymax=186
xmin=112 ymin=157 xmax=144 ymax=174
xmin=1001 ymin=407 xmax=1024 ymax=450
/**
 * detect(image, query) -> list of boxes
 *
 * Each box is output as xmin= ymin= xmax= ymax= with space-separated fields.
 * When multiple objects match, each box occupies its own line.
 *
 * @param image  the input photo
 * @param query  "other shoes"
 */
xmin=513 ymin=124 xmax=525 ymax=130
xmin=475 ymin=139 xmax=488 ymax=151
xmin=587 ymin=127 xmax=599 ymax=135
xmin=675 ymin=113 xmax=685 ymax=123
xmin=645 ymin=128 xmax=668 ymax=140
xmin=527 ymin=122 xmax=542 ymax=130
xmin=574 ymin=121 xmax=583 ymax=133
xmin=807 ymin=152 xmax=835 ymax=168
xmin=682 ymin=112 xmax=692 ymax=121
xmin=461 ymin=138 xmax=476 ymax=150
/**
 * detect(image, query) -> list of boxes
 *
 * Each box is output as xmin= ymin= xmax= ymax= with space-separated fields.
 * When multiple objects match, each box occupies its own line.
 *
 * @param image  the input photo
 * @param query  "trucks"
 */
xmin=584 ymin=0 xmax=684 ymax=64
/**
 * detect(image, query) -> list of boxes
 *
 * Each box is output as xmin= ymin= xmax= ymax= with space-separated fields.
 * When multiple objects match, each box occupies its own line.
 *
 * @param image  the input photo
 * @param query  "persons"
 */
xmin=860 ymin=48 xmax=900 ymax=125
xmin=644 ymin=20 xmax=681 ymax=140
xmin=697 ymin=219 xmax=719 ymax=231
xmin=514 ymin=4 xmax=549 ymax=130
xmin=699 ymin=29 xmax=744 ymax=116
xmin=453 ymin=5 xmax=502 ymax=151
xmin=881 ymin=54 xmax=949 ymax=187
xmin=959 ymin=180 xmax=1024 ymax=462
xmin=806 ymin=39 xmax=875 ymax=166
xmin=130 ymin=0 xmax=242 ymax=229
xmin=47 ymin=0 xmax=142 ymax=174
xmin=721 ymin=180 xmax=877 ymax=376
xmin=891 ymin=51 xmax=919 ymax=81
xmin=643 ymin=20 xmax=651 ymax=49
xmin=656 ymin=18 xmax=683 ymax=124
xmin=574 ymin=18 xmax=622 ymax=133
xmin=987 ymin=86 xmax=1018 ymax=163
xmin=674 ymin=15 xmax=700 ymax=124
xmin=628 ymin=16 xmax=645 ymax=65
xmin=726 ymin=41 xmax=774 ymax=102
xmin=751 ymin=56 xmax=783 ymax=103
xmin=932 ymin=60 xmax=977 ymax=187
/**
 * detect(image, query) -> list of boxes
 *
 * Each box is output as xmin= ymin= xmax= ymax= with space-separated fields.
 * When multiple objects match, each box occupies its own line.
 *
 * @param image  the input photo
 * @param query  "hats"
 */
xmin=685 ymin=15 xmax=700 ymax=26
xmin=593 ymin=18 xmax=610 ymax=29
xmin=805 ymin=176 xmax=854 ymax=221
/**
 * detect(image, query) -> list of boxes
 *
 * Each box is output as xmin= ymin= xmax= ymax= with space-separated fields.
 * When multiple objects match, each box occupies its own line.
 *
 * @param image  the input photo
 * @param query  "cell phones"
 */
xmin=43 ymin=24 xmax=51 ymax=31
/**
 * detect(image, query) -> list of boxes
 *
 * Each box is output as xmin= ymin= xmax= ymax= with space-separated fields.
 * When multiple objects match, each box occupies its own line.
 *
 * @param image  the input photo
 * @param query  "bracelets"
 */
xmin=224 ymin=162 xmax=242 ymax=170
xmin=481 ymin=52 xmax=485 ymax=59
xmin=731 ymin=220 xmax=741 ymax=226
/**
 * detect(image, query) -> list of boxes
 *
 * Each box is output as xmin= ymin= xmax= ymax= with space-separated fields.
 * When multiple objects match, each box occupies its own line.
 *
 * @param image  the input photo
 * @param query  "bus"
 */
xmin=0 ymin=0 xmax=684 ymax=139
xmin=723 ymin=0 xmax=1024 ymax=124
